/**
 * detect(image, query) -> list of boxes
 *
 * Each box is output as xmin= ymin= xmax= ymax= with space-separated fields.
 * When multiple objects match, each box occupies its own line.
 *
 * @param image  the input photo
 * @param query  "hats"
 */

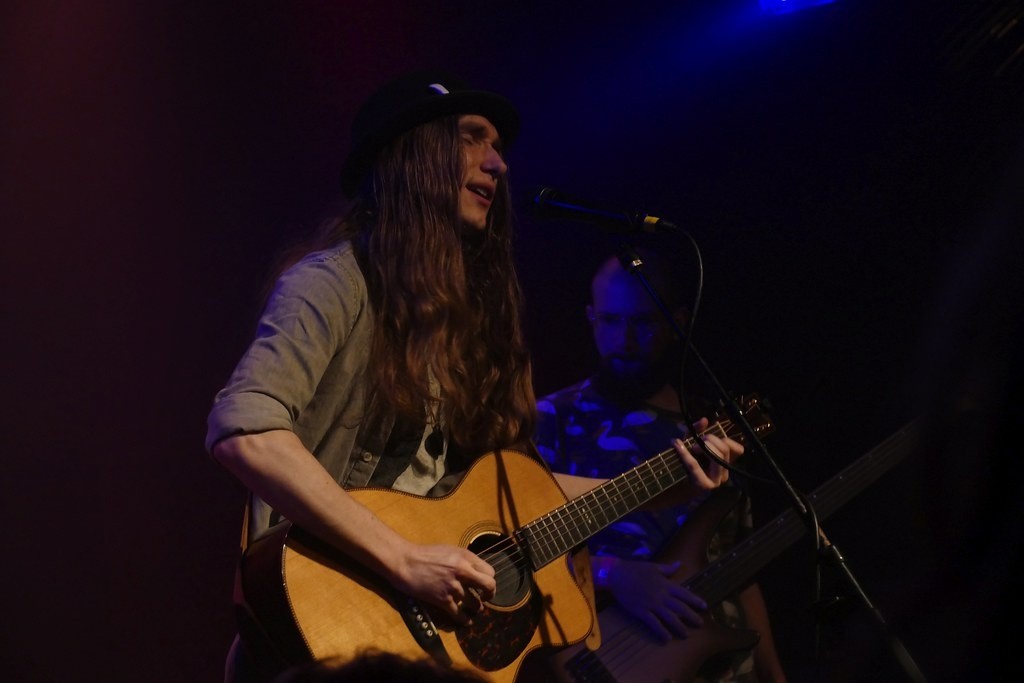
xmin=340 ymin=70 xmax=523 ymax=209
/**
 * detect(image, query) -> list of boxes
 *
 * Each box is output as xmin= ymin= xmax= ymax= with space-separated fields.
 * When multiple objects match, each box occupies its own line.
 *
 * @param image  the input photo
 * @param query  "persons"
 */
xmin=536 ymin=223 xmax=784 ymax=682
xmin=204 ymin=66 xmax=744 ymax=682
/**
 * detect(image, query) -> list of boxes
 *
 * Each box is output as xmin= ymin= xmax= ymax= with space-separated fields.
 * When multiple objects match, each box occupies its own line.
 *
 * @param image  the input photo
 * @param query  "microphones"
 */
xmin=524 ymin=186 xmax=687 ymax=240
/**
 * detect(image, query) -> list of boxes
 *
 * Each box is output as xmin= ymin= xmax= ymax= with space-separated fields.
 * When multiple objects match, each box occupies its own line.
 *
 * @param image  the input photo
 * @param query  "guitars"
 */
xmin=240 ymin=395 xmax=777 ymax=683
xmin=542 ymin=391 xmax=930 ymax=681
